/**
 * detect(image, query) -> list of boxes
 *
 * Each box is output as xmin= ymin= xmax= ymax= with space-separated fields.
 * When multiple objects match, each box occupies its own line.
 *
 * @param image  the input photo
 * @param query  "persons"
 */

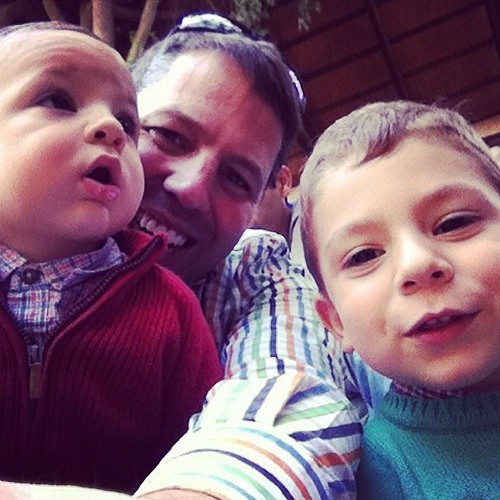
xmin=296 ymin=101 xmax=500 ymax=499
xmin=242 ymin=147 xmax=383 ymax=426
xmin=0 ymin=22 xmax=216 ymax=498
xmin=118 ymin=29 xmax=371 ymax=500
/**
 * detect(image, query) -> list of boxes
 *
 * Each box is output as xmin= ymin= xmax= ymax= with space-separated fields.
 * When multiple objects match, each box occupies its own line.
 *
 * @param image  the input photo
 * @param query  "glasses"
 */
xmin=164 ymin=11 xmax=308 ymax=117
